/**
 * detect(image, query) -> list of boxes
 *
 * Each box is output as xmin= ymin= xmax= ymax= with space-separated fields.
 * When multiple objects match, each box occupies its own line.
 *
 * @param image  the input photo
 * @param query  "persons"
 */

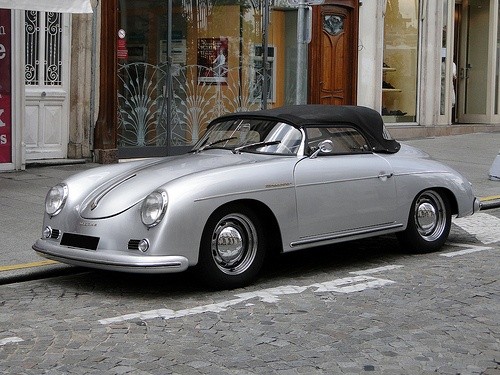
xmin=441 ymin=56 xmax=458 ymax=110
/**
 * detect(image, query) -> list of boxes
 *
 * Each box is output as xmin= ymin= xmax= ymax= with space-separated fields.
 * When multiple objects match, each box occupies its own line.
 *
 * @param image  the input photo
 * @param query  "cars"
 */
xmin=33 ymin=105 xmax=480 ymax=287
